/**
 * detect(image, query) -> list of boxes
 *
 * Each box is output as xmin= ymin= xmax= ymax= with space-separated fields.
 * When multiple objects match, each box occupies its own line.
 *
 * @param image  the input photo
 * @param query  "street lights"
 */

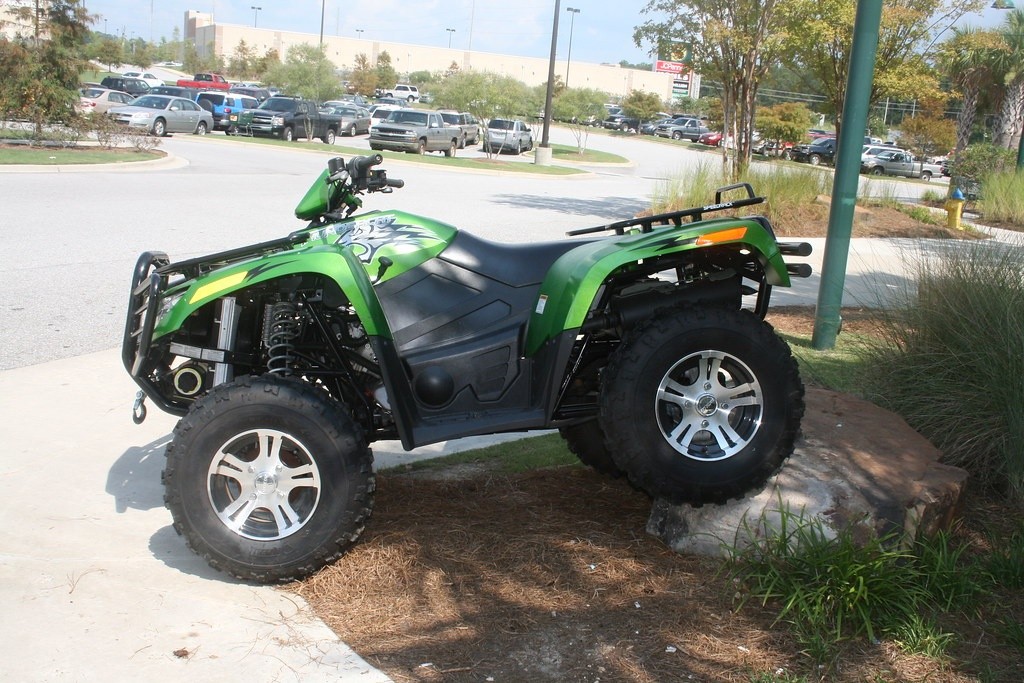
xmin=565 ymin=7 xmax=580 ymax=87
xmin=447 ymin=28 xmax=455 ymax=48
xmin=251 ymin=6 xmax=261 ymax=27
xmin=356 ymin=30 xmax=364 ymax=39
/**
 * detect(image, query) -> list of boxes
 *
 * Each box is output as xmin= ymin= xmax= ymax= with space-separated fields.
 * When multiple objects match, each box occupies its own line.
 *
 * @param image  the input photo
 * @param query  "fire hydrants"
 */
xmin=944 ymin=188 xmax=966 ymax=231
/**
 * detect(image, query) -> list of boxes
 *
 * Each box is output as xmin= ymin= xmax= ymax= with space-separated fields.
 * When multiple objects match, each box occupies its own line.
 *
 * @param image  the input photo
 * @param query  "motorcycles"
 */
xmin=121 ymin=156 xmax=840 ymax=581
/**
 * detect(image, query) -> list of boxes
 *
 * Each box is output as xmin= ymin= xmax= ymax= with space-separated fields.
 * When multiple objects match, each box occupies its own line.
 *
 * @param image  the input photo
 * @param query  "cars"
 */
xmin=933 ymin=152 xmax=953 ymax=177
xmin=70 ymin=88 xmax=137 ymax=121
xmin=641 ymin=118 xmax=674 ymax=137
xmin=332 ymin=107 xmax=370 ymax=137
xmin=106 ymin=94 xmax=214 ymax=137
xmin=421 ymin=92 xmax=435 ymax=103
xmin=340 ymin=94 xmax=371 ymax=109
xmin=370 ymin=106 xmax=402 ymax=130
xmin=699 ymin=132 xmax=726 ymax=147
xmin=319 ymin=100 xmax=355 ymax=112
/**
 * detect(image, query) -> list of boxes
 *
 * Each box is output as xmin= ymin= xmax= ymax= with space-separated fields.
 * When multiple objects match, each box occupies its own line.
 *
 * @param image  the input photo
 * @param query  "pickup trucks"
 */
xmin=177 ymin=73 xmax=231 ymax=92
xmin=239 ymin=97 xmax=342 ymax=145
xmin=768 ymin=134 xmax=830 ymax=161
xmin=369 ymin=110 xmax=461 ymax=156
xmin=657 ymin=117 xmax=710 ymax=142
xmin=864 ymin=150 xmax=942 ymax=181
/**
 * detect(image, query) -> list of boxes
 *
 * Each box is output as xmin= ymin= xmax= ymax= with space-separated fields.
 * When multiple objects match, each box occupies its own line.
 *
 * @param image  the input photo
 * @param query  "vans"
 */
xmin=229 ymin=88 xmax=271 ymax=102
xmin=862 ymin=144 xmax=908 ymax=167
xmin=141 ymin=86 xmax=199 ymax=102
xmin=101 ymin=77 xmax=150 ymax=96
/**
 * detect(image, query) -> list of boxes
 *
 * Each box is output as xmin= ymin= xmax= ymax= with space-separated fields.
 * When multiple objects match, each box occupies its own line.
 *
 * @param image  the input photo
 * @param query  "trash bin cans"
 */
xmin=534 ymin=147 xmax=553 ymax=165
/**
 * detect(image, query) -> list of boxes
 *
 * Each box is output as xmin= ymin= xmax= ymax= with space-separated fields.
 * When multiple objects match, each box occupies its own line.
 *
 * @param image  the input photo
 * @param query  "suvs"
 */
xmin=602 ymin=109 xmax=643 ymax=132
xmin=789 ymin=137 xmax=838 ymax=166
xmin=483 ymin=118 xmax=533 ymax=153
xmin=195 ymin=91 xmax=260 ymax=136
xmin=381 ymin=84 xmax=420 ymax=103
xmin=437 ymin=110 xmax=481 ymax=149
